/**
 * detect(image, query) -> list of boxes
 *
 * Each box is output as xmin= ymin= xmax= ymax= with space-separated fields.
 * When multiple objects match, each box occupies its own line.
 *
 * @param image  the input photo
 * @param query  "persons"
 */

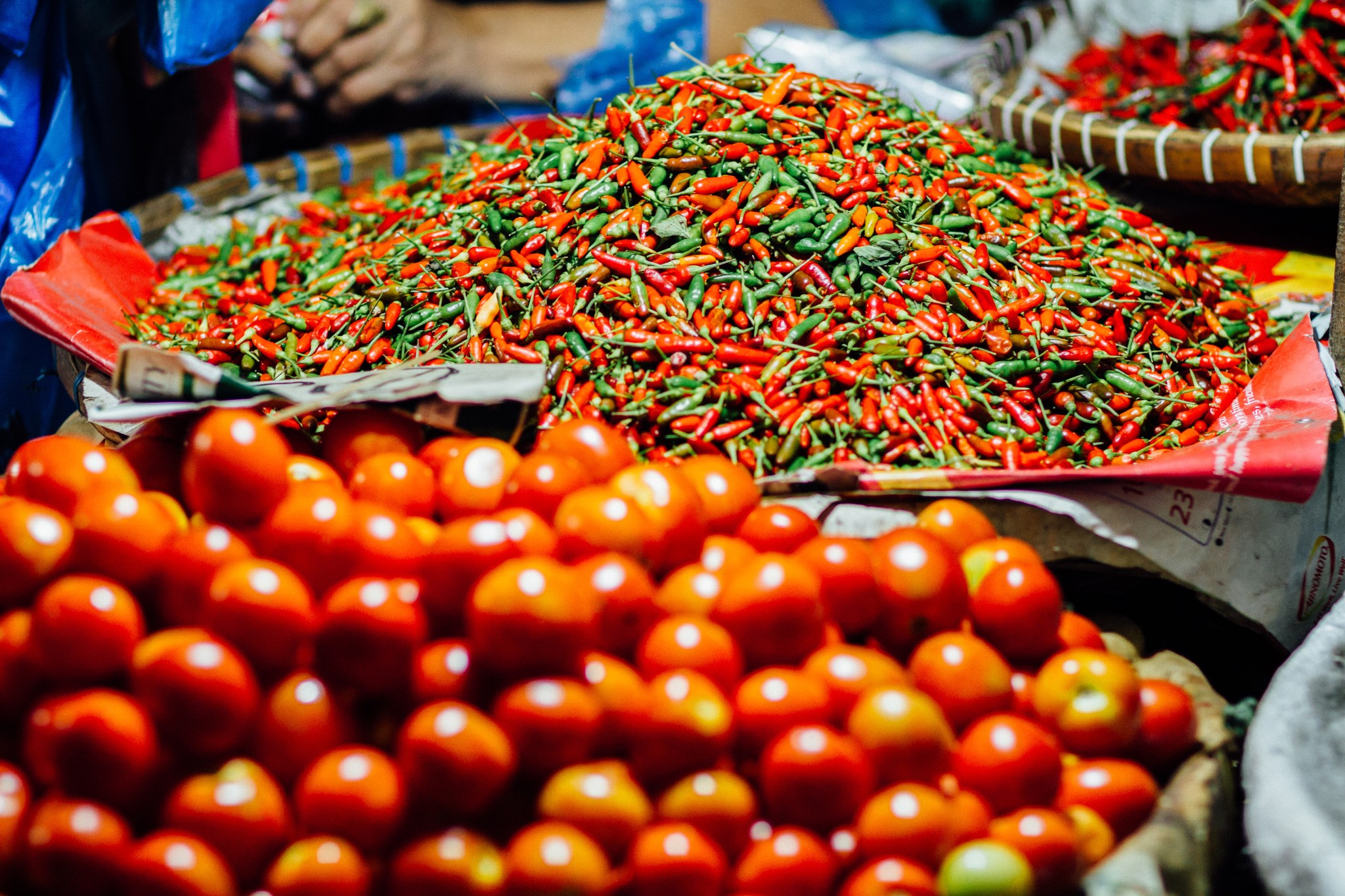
xmin=231 ymin=0 xmax=839 ymax=124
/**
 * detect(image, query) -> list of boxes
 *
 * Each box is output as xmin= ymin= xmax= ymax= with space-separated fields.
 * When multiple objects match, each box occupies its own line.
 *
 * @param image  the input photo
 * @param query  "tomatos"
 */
xmin=0 ymin=408 xmax=1199 ymax=896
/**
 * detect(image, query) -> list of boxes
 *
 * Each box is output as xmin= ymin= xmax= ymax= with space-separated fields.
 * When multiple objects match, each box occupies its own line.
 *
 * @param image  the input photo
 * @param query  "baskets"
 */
xmin=976 ymin=0 xmax=1345 ymax=202
xmin=49 ymin=124 xmax=508 ymax=439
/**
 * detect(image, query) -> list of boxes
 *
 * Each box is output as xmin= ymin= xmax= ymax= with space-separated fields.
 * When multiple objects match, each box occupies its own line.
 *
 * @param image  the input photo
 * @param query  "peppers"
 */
xmin=114 ymin=25 xmax=1289 ymax=472
xmin=1032 ymin=0 xmax=1345 ymax=140
